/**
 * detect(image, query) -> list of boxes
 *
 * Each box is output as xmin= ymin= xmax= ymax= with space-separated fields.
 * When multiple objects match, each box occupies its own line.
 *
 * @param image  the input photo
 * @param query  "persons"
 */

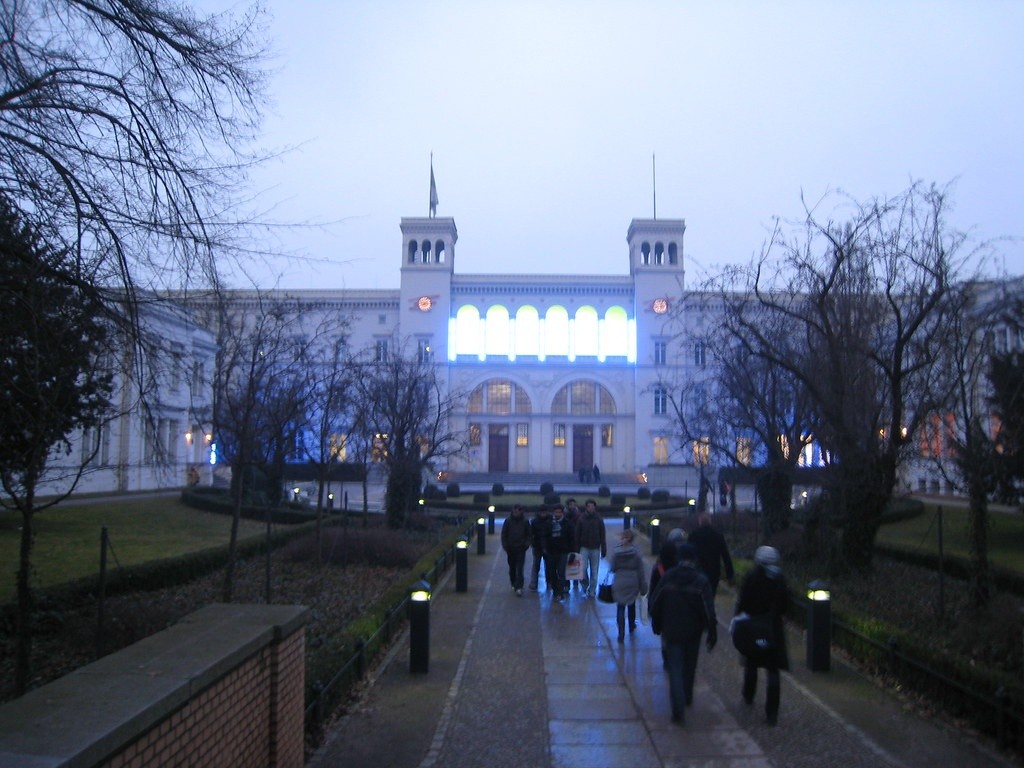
xmin=609 ymin=529 xmax=648 ymax=642
xmin=700 ymin=475 xmax=729 ymax=507
xmin=187 ymin=466 xmax=200 ymax=487
xmin=650 ymin=511 xmax=735 ymax=672
xmin=578 ymin=464 xmax=600 ymax=484
xmin=501 ymin=498 xmax=606 ymax=602
xmin=731 ymin=546 xmax=793 ymax=725
xmin=651 ymin=544 xmax=718 ymax=724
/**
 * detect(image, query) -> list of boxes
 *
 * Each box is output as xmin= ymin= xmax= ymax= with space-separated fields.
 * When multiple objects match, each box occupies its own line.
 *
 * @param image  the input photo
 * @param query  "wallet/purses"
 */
xmin=568 ymin=553 xmax=575 ymax=563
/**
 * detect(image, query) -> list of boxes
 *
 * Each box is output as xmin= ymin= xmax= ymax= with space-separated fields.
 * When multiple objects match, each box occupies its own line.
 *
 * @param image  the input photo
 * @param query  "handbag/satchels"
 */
xmin=597 ymin=571 xmax=614 ymax=603
xmin=564 ymin=552 xmax=584 ymax=580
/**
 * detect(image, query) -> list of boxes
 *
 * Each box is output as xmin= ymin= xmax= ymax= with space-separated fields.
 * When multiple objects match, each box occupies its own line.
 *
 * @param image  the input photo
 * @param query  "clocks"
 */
xmin=418 ymin=296 xmax=431 ymax=312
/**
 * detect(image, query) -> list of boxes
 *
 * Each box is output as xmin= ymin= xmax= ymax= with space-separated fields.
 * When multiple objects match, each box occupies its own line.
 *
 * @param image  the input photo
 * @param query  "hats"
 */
xmin=679 ymin=543 xmax=696 ymax=560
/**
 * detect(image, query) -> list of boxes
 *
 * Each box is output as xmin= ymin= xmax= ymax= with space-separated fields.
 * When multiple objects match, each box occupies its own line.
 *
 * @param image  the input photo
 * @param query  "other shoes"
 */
xmin=669 ymin=705 xmax=686 ymax=723
xmin=515 ymin=587 xmax=522 ymax=596
xmin=563 ymin=585 xmax=569 ymax=593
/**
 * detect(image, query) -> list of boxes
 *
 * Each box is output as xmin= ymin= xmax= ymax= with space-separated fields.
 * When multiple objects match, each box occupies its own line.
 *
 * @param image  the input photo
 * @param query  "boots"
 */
xmin=617 ymin=616 xmax=625 ymax=642
xmin=627 ymin=606 xmax=637 ymax=633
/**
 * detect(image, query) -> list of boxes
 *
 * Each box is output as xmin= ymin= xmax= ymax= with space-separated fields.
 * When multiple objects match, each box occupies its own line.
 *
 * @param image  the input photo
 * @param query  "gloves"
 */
xmin=706 ymin=628 xmax=718 ymax=650
xmin=652 ymin=617 xmax=662 ymax=635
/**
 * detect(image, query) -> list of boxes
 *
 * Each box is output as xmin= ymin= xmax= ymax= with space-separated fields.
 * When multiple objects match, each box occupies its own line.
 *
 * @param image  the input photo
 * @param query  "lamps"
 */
xmin=805 ymin=577 xmax=831 ymax=602
xmin=477 ymin=517 xmax=485 ymax=525
xmin=688 ymin=498 xmax=695 ymax=505
xmin=650 ymin=515 xmax=660 ymax=526
xmin=407 ymin=577 xmax=431 ymax=602
xmin=653 ymin=298 xmax=668 ymax=314
xmin=454 ymin=533 xmax=468 ymax=549
xmin=488 ymin=505 xmax=495 ymax=512
xmin=623 ymin=504 xmax=631 ymax=513
